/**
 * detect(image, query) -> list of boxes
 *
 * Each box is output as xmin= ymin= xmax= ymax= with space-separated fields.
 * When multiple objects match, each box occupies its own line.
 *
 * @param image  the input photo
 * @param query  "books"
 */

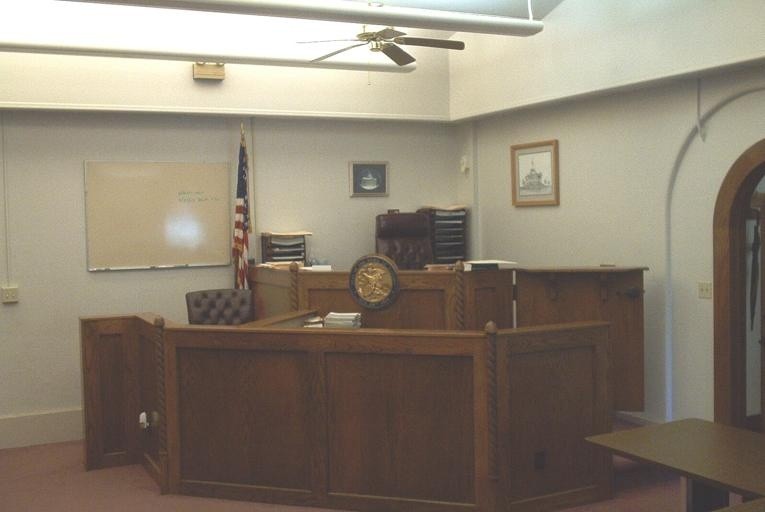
xmin=322 ymin=312 xmax=362 ymax=331
xmin=463 ymin=260 xmax=518 ymax=272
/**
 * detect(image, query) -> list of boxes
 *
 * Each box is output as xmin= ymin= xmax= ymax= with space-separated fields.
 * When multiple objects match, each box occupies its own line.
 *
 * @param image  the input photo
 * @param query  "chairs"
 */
xmin=185 ymin=288 xmax=253 ymax=325
xmin=375 ymin=212 xmax=436 ymax=270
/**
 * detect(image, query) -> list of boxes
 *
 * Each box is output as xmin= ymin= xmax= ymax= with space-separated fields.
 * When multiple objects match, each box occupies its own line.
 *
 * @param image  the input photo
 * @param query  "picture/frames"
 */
xmin=511 ymin=138 xmax=560 ymax=207
xmin=348 ymin=160 xmax=389 ymax=197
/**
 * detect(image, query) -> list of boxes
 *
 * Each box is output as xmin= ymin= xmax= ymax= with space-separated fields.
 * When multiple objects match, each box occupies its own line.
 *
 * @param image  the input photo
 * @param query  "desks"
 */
xmin=581 ymin=417 xmax=765 ymax=500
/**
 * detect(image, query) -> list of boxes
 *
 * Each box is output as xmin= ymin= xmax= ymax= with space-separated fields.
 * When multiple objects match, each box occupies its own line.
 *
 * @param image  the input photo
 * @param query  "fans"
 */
xmin=296 ymin=3 xmax=466 ymax=67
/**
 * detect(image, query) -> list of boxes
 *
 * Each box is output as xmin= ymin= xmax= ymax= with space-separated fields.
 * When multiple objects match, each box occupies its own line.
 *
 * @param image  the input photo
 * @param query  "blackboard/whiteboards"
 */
xmin=84 ymin=159 xmax=233 ymax=272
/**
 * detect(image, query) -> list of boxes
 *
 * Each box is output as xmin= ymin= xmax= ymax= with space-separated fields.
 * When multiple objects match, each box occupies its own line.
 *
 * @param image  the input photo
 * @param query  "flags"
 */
xmin=232 ymin=122 xmax=252 ymax=290
